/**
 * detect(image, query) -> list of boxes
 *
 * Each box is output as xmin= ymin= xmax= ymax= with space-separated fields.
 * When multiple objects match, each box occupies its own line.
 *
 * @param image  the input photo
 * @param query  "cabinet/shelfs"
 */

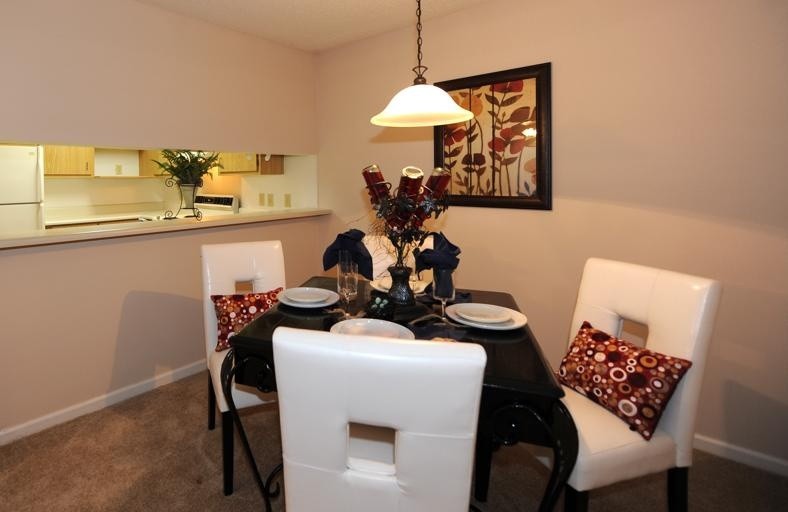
xmin=44 ymin=144 xmax=95 ymax=178
xmin=94 ymin=147 xmax=145 ymax=178
xmin=218 ymin=153 xmax=284 ymax=177
xmin=138 ymin=151 xmax=174 ymax=177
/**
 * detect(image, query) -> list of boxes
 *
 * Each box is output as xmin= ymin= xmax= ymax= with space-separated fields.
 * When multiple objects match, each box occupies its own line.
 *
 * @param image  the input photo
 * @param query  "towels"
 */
xmin=412 ymin=230 xmax=461 ymax=297
xmin=323 ymin=230 xmax=375 ymax=282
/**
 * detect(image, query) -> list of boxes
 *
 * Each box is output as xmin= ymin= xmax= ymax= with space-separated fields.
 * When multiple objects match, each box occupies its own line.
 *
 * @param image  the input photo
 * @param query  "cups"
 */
xmin=337 ymin=250 xmax=359 ymax=302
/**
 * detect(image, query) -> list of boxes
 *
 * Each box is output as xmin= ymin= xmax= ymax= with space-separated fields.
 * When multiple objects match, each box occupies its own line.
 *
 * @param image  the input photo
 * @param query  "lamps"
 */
xmin=369 ymin=0 xmax=474 ymax=127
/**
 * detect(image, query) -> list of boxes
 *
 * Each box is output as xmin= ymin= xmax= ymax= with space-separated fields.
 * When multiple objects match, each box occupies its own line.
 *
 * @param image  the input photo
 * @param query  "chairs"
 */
xmin=202 ymin=241 xmax=287 ymax=497
xmin=361 ymin=232 xmax=434 ymax=292
xmin=519 ymin=257 xmax=724 ymax=512
xmin=271 ymin=325 xmax=488 ymax=512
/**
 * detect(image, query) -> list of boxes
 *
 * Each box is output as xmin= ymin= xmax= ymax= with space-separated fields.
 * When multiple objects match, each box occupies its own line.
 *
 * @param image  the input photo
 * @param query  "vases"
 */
xmin=387 ymin=266 xmax=416 ymax=307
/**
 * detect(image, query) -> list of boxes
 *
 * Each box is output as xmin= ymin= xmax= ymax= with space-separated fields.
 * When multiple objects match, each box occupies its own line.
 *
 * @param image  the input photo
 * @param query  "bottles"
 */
xmin=361 ymin=164 xmax=451 ymax=231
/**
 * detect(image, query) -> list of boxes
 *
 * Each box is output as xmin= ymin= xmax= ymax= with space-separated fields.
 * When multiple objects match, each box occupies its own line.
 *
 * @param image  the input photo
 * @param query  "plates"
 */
xmin=276 ymin=280 xmax=527 ymax=340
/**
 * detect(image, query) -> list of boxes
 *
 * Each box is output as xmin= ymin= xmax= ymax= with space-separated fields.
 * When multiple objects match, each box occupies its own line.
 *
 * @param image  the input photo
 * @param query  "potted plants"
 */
xmin=150 ymin=149 xmax=225 ymax=207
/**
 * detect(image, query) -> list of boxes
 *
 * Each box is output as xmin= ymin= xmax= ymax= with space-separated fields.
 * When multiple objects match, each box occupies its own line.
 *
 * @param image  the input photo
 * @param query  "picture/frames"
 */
xmin=433 ymin=61 xmax=552 ymax=210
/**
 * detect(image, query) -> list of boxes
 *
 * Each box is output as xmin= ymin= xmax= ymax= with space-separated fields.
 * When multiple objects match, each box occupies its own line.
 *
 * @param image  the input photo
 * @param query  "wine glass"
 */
xmin=433 ymin=270 xmax=455 ymax=317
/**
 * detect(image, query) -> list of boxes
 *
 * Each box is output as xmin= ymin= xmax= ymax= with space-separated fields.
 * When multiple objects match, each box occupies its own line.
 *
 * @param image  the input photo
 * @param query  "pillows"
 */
xmin=554 ymin=321 xmax=693 ymax=441
xmin=209 ymin=287 xmax=283 ymax=353
